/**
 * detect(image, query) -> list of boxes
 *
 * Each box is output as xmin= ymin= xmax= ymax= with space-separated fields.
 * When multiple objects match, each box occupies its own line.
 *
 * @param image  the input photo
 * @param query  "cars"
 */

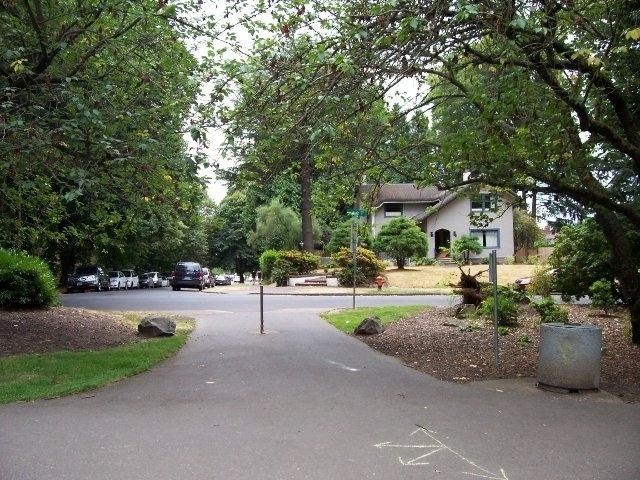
xmin=67 ymin=261 xmax=245 ymax=292
xmin=513 ymin=268 xmax=559 ymax=293
